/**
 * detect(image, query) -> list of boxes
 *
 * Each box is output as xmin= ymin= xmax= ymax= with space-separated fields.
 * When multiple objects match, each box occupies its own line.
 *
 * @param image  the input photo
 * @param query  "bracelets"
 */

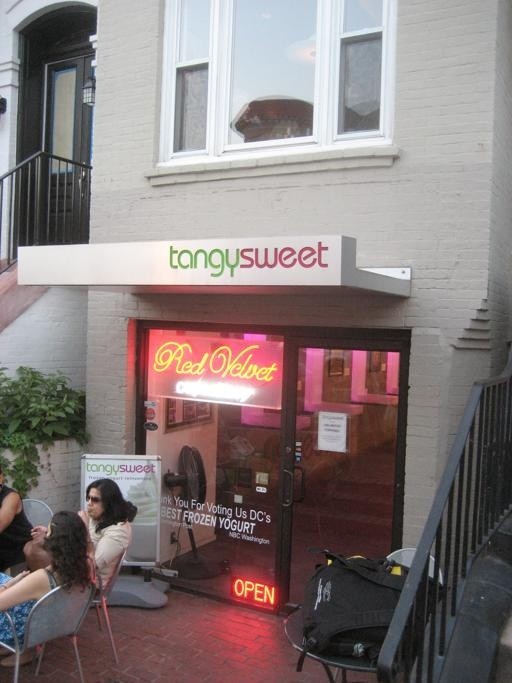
xmin=1 ymin=584 xmax=8 ymax=589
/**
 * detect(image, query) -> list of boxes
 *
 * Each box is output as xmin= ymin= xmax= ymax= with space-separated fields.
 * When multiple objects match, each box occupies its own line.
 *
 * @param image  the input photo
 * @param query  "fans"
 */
xmin=164 ymin=446 xmax=219 ymax=580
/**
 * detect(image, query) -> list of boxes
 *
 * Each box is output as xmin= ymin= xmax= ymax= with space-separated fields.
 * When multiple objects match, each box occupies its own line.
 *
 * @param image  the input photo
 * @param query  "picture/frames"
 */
xmin=162 ymin=399 xmax=215 ymax=435
xmin=369 ymin=351 xmax=381 ymax=373
xmin=329 ymin=351 xmax=344 ymax=376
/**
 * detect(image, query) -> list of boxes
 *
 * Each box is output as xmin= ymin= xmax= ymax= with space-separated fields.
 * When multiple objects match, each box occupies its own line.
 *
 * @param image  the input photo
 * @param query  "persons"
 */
xmin=24 ymin=477 xmax=138 ymax=594
xmin=1 ymin=510 xmax=92 ymax=645
xmin=1 ymin=470 xmax=35 ymax=573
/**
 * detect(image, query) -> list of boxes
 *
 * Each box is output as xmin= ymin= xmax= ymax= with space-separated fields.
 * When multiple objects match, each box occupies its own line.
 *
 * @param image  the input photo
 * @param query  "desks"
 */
xmin=284 ymin=607 xmax=377 ymax=683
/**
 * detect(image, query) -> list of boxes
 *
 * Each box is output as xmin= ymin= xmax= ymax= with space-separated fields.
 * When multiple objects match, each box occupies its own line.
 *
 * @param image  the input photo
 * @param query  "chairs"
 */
xmin=385 ymin=548 xmax=443 ymax=585
xmin=0 ymin=498 xmax=127 ymax=683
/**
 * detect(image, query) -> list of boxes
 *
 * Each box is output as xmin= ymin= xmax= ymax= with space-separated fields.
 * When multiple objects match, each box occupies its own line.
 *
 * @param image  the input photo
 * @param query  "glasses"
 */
xmin=86 ymin=495 xmax=101 ymax=502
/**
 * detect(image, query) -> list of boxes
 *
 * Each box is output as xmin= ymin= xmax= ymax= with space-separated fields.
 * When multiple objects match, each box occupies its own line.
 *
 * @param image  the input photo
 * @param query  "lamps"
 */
xmin=82 ymin=76 xmax=95 ymax=105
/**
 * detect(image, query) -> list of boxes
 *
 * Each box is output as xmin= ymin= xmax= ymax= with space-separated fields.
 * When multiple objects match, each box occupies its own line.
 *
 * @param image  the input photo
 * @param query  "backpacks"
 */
xmin=297 ymin=547 xmax=442 ymax=669
